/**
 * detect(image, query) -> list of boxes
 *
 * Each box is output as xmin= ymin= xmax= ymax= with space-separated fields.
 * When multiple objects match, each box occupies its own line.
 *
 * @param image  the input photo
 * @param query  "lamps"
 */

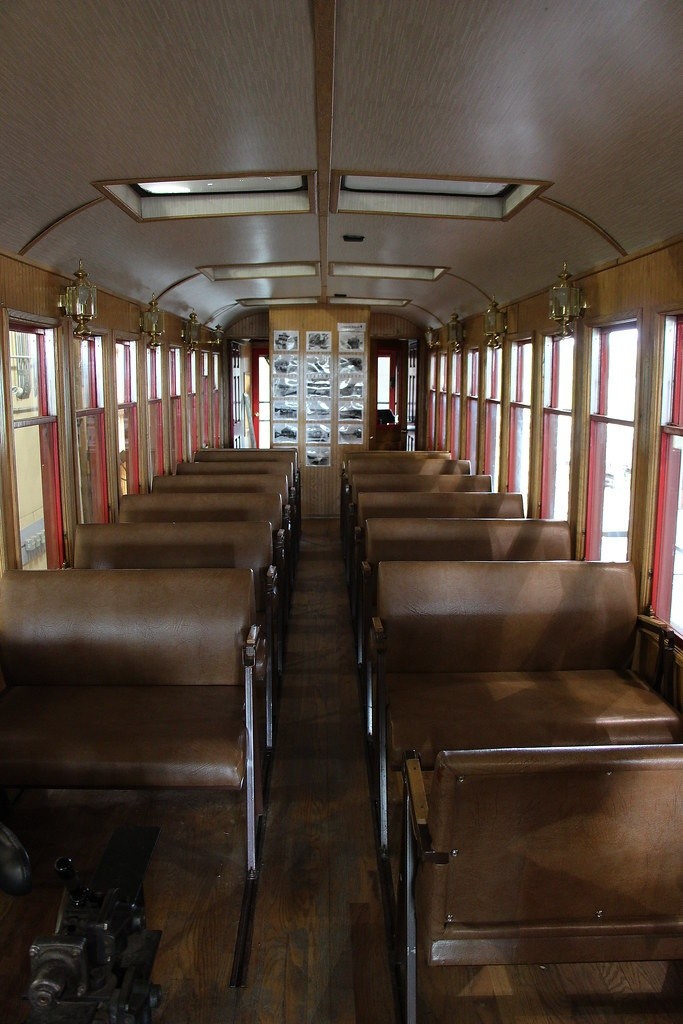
xmin=140 ymin=292 xmax=166 ymax=346
xmin=547 ymin=260 xmax=588 ymax=338
xmin=446 ymin=313 xmax=464 ymax=353
xmin=180 ymin=308 xmax=202 ymax=352
xmin=58 ymin=258 xmax=99 ymax=341
xmin=484 ymin=294 xmax=506 ymax=348
xmin=425 ymin=326 xmax=440 ymax=348
xmin=207 ymin=323 xmax=225 ymax=345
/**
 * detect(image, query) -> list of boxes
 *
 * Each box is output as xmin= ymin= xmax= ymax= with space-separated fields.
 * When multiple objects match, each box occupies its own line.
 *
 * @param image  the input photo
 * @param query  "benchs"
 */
xmin=1 ymin=450 xmax=306 ymax=987
xmin=341 ymin=449 xmax=683 ymax=1023
